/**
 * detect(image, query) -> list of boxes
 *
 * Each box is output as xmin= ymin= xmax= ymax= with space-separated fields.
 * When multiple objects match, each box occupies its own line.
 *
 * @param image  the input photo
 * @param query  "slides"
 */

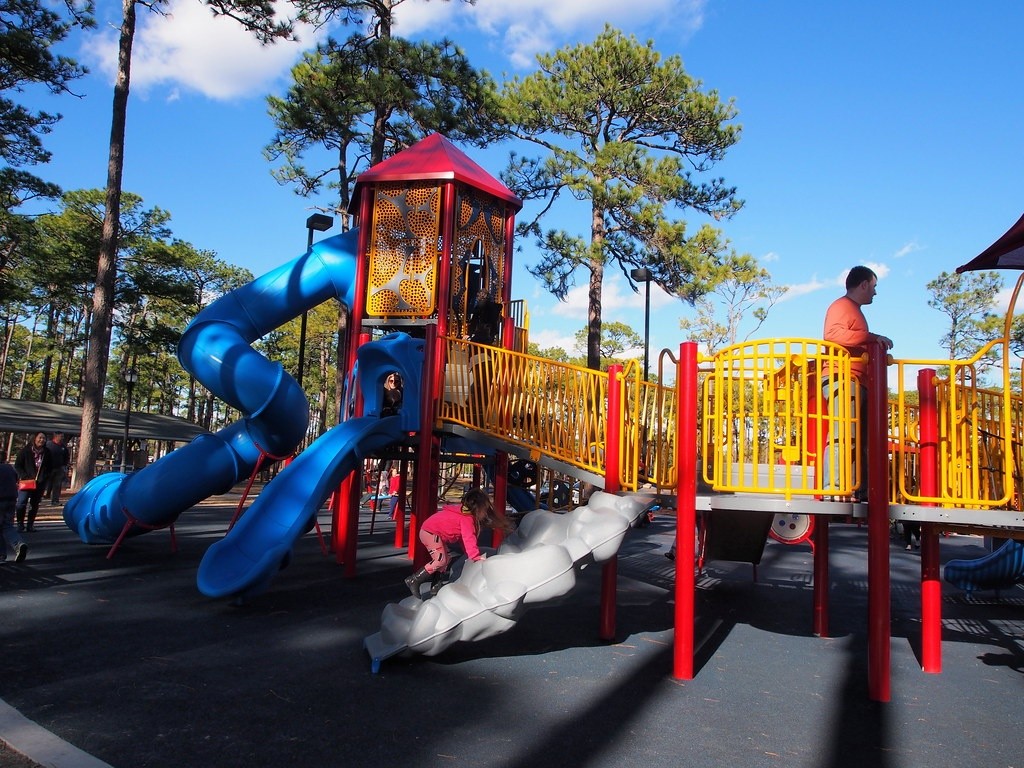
xmin=198 ymin=413 xmax=403 ymax=598
xmin=65 ymin=228 xmax=361 ymax=544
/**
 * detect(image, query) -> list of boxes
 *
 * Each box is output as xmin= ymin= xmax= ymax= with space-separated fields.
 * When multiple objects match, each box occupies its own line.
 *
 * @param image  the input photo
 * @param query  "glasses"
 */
xmin=389 ymin=380 xmax=400 ymax=384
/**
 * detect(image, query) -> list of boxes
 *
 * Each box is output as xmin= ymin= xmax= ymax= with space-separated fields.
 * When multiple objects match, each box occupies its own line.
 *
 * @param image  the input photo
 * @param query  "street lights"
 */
xmin=631 ymin=268 xmax=653 ymax=382
xmin=298 ymin=213 xmax=335 ymax=391
xmin=120 ymin=364 xmax=139 ymax=472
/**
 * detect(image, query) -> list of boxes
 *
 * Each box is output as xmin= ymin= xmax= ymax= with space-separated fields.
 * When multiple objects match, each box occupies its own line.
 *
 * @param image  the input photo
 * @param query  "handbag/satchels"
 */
xmin=18 ymin=480 xmax=36 ymax=491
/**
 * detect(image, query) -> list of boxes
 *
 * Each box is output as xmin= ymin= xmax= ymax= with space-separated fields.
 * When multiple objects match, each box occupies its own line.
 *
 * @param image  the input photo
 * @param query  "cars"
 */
xmin=540 ymin=480 xmax=555 ymax=498
xmin=572 ymin=481 xmax=588 ymax=505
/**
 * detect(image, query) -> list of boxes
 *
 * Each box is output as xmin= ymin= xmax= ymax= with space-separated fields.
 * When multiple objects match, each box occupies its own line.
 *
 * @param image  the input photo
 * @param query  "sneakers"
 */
xmin=0 ymin=553 xmax=8 ymax=562
xmin=15 ymin=542 xmax=27 ymax=562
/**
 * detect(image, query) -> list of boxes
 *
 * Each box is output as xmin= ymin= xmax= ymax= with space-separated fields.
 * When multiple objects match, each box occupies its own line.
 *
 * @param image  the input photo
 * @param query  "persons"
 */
xmin=0 ymin=449 xmax=28 ymax=565
xmin=358 ymin=467 xmax=390 ymax=512
xmin=42 ymin=430 xmax=72 ymax=507
xmin=100 ymin=437 xmax=150 ymax=476
xmin=13 ymin=431 xmax=52 ymax=532
xmin=817 ymin=265 xmax=894 ymax=504
xmin=402 ymin=487 xmax=490 ymax=600
xmin=380 ymin=372 xmax=408 ymax=416
xmin=385 ymin=468 xmax=401 ymax=519
xmin=667 ymin=441 xmax=723 ymax=560
xmin=897 ymin=475 xmax=921 ymax=551
xmin=467 ymin=298 xmax=503 ymax=423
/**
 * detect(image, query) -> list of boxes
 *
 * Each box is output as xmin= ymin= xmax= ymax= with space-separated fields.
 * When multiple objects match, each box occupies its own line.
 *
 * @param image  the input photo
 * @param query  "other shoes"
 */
xmin=50 ymin=500 xmax=62 ymax=506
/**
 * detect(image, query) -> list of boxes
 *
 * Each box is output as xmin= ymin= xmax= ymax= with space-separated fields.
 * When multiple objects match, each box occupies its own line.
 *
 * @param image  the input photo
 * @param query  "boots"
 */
xmin=405 ymin=567 xmax=430 ymax=599
xmin=16 ymin=505 xmax=26 ymax=532
xmin=27 ymin=506 xmax=40 ymax=532
xmin=431 ymin=571 xmax=442 ymax=595
